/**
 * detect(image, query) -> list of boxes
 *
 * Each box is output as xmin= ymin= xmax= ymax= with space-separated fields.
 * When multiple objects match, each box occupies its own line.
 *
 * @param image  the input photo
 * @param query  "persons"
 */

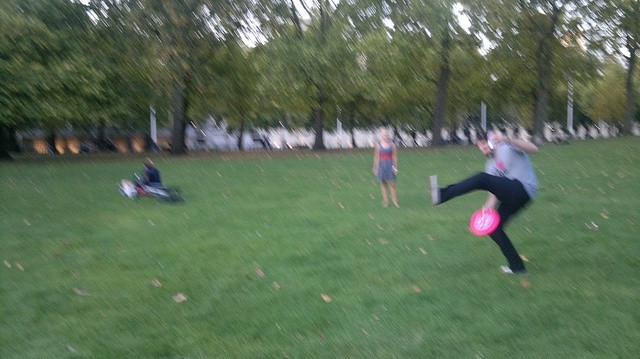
xmin=372 ymin=128 xmax=400 ymax=209
xmin=141 ymin=156 xmax=166 ymax=190
xmin=429 ymin=129 xmax=539 ymax=275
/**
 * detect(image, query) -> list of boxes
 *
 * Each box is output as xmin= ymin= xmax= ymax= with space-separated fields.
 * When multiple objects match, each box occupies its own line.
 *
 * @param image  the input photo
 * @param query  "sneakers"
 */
xmin=501 ymin=266 xmax=527 ymax=273
xmin=430 ymin=175 xmax=441 ymax=205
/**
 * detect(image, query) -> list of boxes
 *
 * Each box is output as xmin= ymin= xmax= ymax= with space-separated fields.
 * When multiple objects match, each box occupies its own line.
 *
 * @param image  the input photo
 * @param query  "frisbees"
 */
xmin=469 ymin=207 xmax=500 ymax=236
